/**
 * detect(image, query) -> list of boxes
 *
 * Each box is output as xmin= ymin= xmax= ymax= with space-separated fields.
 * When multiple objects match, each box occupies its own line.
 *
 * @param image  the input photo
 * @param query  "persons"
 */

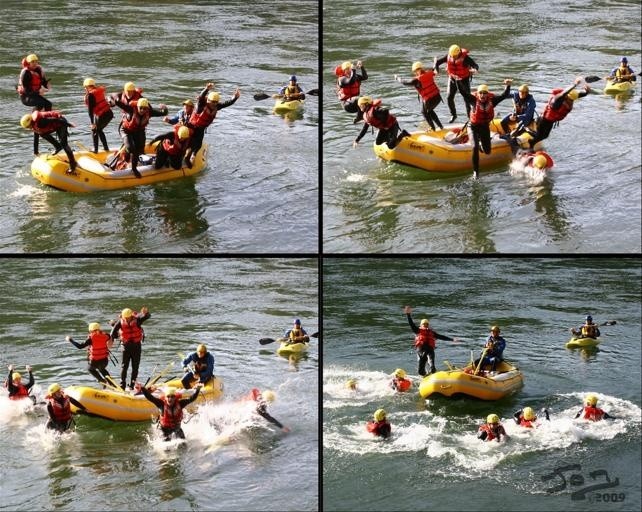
xmin=387 ymin=368 xmax=410 ymax=390
xmin=182 ymin=344 xmax=214 ymax=391
xmin=5 ymin=365 xmax=34 ymax=401
xmin=214 ymin=390 xmax=289 ymax=427
xmin=335 ymin=44 xmax=591 ymax=181
xmin=112 ymin=306 xmax=152 ymax=389
xmin=476 ymin=324 xmax=506 ymax=372
xmin=478 ymin=412 xmax=512 ymax=443
xmin=67 ymin=321 xmax=117 ymax=387
xmin=572 ymin=314 xmax=603 ymax=337
xmin=281 ymin=75 xmax=304 ymax=101
xmin=15 ymin=55 xmax=52 ymax=113
xmin=45 ymin=384 xmax=84 ymax=433
xmin=20 ymin=110 xmax=78 ymax=174
xmin=132 ymin=380 xmax=202 ymax=445
xmin=609 ymin=58 xmax=636 ymax=83
xmin=365 ymin=410 xmax=392 ymax=439
xmin=345 ymin=381 xmax=361 ymax=391
xmin=285 ymin=320 xmax=310 ymax=344
xmin=404 ymin=306 xmax=459 ymax=372
xmin=82 ymin=77 xmax=240 ymax=179
xmin=514 ymin=408 xmax=552 ymax=428
xmin=576 ymin=395 xmax=624 ymax=422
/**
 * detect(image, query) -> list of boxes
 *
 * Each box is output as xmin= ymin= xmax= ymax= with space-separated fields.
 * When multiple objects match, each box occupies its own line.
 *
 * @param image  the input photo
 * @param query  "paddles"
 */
xmin=449 ymin=130 xmax=467 ymax=144
xmin=585 ymin=73 xmax=642 ymax=83
xmin=108 ymin=145 xmax=126 ymax=167
xmin=582 ymin=321 xmax=616 ymax=326
xmin=254 ymin=89 xmax=319 ymax=101
xmin=259 ymin=332 xmax=318 ymax=345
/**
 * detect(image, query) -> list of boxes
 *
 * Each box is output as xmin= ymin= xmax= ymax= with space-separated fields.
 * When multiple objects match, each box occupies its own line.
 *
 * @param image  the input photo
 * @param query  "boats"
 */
xmin=418 ymin=360 xmax=526 ymax=403
xmin=566 ymin=337 xmax=601 ymax=351
xmin=603 ymin=75 xmax=635 ymax=99
xmin=277 ymin=335 xmax=311 ymax=358
xmin=373 ymin=121 xmax=544 ymax=174
xmin=273 ymin=88 xmax=304 ymax=114
xmin=28 ymin=140 xmax=215 ymax=193
xmin=59 ymin=375 xmax=226 ymax=423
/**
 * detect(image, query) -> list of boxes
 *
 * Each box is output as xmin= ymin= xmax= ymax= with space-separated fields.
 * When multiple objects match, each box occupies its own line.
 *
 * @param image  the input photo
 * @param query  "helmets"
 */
xmin=523 ymin=407 xmax=534 ymax=420
xmin=165 ymin=389 xmax=176 ymax=396
xmin=395 ymin=369 xmax=405 ymax=378
xmin=289 ymin=75 xmax=297 ymax=81
xmin=358 ymin=96 xmax=372 ymax=105
xmin=585 ymin=316 xmax=592 ymax=320
xmin=478 ymin=85 xmax=488 ymax=92
xmin=449 ymin=45 xmax=460 ymax=56
xmin=122 ymin=308 xmax=132 ymax=317
xmin=294 ymin=319 xmax=301 ymax=324
xmin=534 ymin=155 xmax=546 ymax=168
xmin=20 ymin=115 xmax=32 ymax=128
xmin=621 ymin=58 xmax=628 ymax=62
xmin=27 ymin=54 xmax=38 ymax=62
xmin=567 ymin=89 xmax=578 ymax=100
xmin=412 ymin=62 xmax=422 ymax=71
xmin=137 ymin=98 xmax=148 ymax=107
xmin=342 ymin=62 xmax=351 ymax=70
xmin=89 ymin=323 xmax=100 ymax=330
xmin=374 ymin=409 xmax=385 ymax=421
xmin=421 ymin=319 xmax=429 ymax=324
xmin=586 ymin=396 xmax=597 ymax=405
xmin=125 ymin=83 xmax=135 ymax=91
xmin=177 ymin=126 xmax=189 ymax=139
xmin=48 ymin=384 xmax=61 ymax=396
xmin=12 ymin=373 xmax=21 ymax=381
xmin=487 ymin=414 xmax=499 ymax=424
xmin=83 ymin=79 xmax=95 ymax=85
xmin=208 ymin=92 xmax=219 ymax=101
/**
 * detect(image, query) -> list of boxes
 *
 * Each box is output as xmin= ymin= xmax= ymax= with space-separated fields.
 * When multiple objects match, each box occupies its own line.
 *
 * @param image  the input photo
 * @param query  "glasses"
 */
xmin=479 ymin=91 xmax=488 ymax=95
xmin=167 ymin=396 xmax=174 ymax=398
xmin=167 ymin=395 xmax=175 ymax=398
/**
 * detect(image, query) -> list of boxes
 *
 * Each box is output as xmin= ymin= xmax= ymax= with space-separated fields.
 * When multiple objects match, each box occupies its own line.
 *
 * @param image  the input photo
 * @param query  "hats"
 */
xmin=197 ymin=344 xmax=206 ymax=353
xmin=519 ymin=85 xmax=528 ymax=92
xmin=491 ymin=326 xmax=499 ymax=331
xmin=183 ymin=100 xmax=193 ymax=106
